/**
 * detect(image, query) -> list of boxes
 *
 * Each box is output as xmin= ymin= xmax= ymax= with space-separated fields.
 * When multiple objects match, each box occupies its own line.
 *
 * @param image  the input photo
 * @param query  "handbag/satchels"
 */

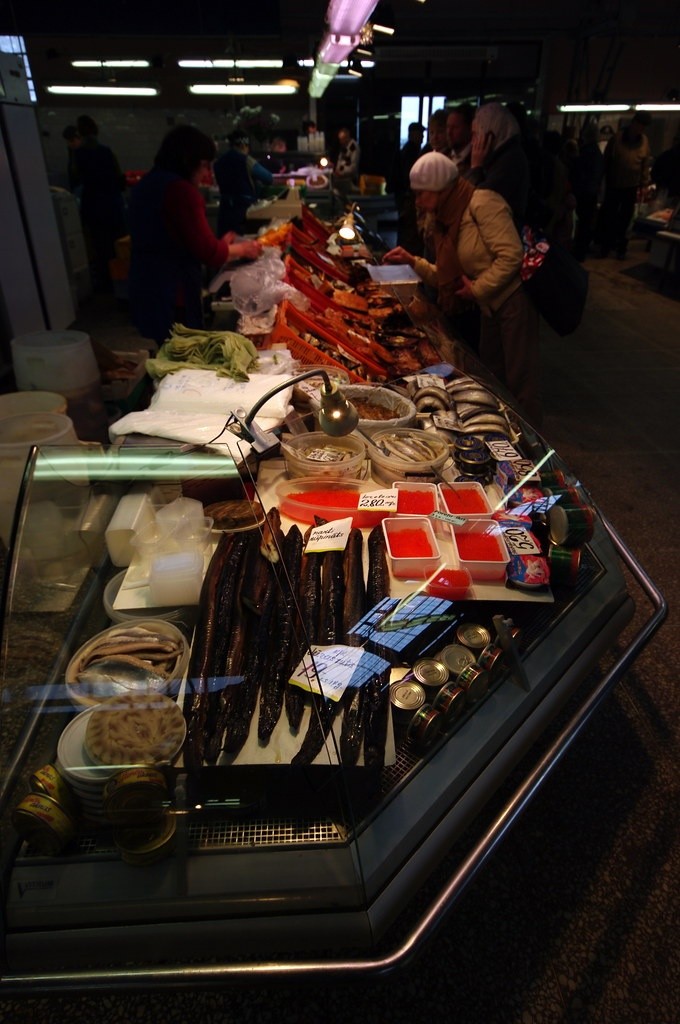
xmin=470 ymin=186 xmax=589 ymax=337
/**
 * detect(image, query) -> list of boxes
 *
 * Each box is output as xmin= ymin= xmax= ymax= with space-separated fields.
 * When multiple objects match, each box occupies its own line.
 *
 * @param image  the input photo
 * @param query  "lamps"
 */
xmin=225 ymin=367 xmax=361 ymax=459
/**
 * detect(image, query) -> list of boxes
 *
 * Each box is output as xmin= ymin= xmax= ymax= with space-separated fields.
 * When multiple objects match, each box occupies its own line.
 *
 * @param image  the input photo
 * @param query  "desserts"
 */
xmin=83 ymin=689 xmax=187 ymax=773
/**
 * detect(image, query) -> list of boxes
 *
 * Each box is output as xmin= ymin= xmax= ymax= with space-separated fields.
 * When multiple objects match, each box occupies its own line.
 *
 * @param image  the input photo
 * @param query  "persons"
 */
xmin=63 ymin=116 xmax=360 ymax=344
xmin=397 ymin=101 xmax=651 ymax=429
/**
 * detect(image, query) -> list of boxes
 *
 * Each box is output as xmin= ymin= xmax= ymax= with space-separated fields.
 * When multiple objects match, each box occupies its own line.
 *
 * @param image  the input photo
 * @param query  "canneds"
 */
xmin=102 ymin=766 xmax=177 ymax=865
xmin=451 ymin=436 xmax=493 ymax=489
xmin=538 ymin=469 xmax=596 ymax=582
xmin=387 ymin=623 xmax=526 ymax=741
xmin=11 ymin=763 xmax=74 ymax=855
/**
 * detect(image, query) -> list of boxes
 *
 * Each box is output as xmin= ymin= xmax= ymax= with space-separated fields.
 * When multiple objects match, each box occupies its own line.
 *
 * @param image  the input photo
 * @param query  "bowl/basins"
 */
xmin=392 ymin=482 xmax=439 ymax=529
xmin=424 ymin=564 xmax=473 ymax=601
xmin=450 ymin=519 xmax=511 ymax=580
xmin=276 ymin=477 xmax=391 ymax=528
xmin=368 ymin=428 xmax=449 ymax=486
xmin=382 ymin=518 xmax=441 ymax=578
xmin=103 ymin=494 xmax=214 ymax=625
xmin=438 ymin=482 xmax=494 ymax=531
xmin=283 ymin=430 xmax=365 ymax=480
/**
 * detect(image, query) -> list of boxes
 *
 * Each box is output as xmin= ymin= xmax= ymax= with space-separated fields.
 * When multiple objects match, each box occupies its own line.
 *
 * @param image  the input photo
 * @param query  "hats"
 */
xmin=410 ymin=149 xmax=457 ymax=191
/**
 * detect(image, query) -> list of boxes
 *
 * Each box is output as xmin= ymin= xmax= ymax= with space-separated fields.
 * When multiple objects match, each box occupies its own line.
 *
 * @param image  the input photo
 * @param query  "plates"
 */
xmin=55 ymin=704 xmax=186 ymax=823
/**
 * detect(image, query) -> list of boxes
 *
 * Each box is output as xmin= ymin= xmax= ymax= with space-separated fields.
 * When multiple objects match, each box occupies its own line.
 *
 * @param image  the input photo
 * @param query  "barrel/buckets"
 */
xmin=12 ymin=333 xmax=100 ymax=397
xmin=0 ymin=391 xmax=67 ymax=418
xmin=0 ymin=412 xmax=94 ymax=560
